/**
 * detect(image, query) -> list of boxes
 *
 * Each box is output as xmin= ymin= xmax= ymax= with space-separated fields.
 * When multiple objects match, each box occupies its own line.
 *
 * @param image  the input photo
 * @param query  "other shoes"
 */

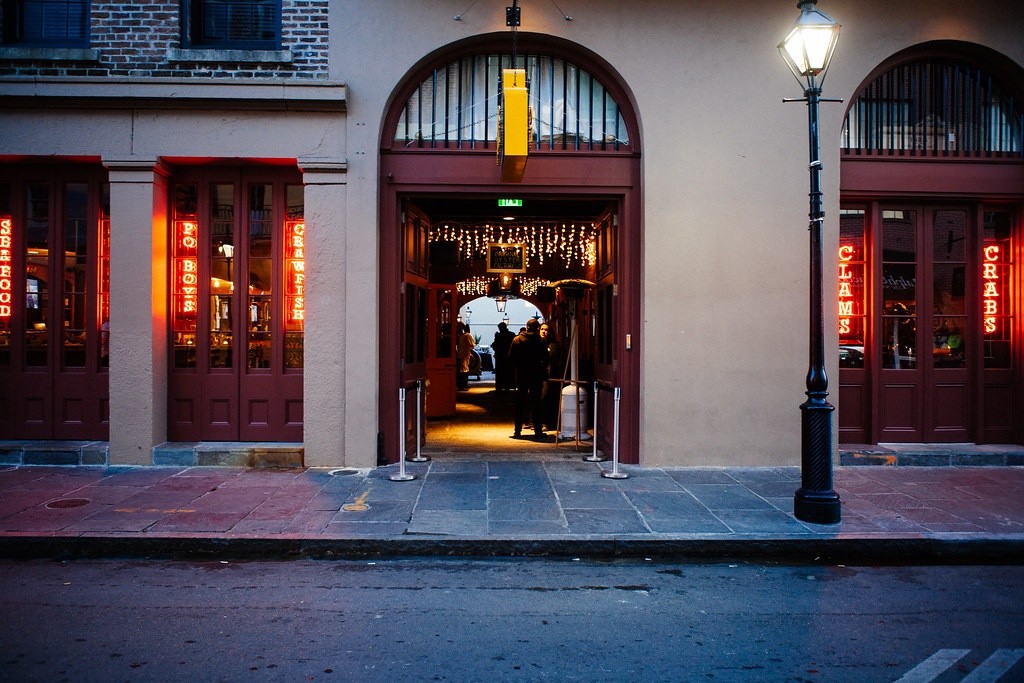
xmin=535 ymin=433 xmax=547 ymax=440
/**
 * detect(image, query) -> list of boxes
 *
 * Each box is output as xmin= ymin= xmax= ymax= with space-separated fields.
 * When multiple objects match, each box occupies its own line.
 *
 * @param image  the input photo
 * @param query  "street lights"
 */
xmin=777 ymin=0 xmax=851 ymax=526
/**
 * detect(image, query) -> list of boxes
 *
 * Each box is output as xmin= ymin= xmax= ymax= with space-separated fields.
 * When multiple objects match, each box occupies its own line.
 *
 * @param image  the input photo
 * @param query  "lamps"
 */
xmin=466 ymin=307 xmax=472 ymax=318
xmin=495 ymin=296 xmax=507 ymax=312
xmin=503 ymin=312 xmax=510 ymax=326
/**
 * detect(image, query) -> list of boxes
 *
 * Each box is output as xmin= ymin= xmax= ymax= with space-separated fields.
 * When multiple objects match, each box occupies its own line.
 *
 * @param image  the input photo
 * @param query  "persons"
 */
xmin=456 ymin=319 xmax=560 ymax=438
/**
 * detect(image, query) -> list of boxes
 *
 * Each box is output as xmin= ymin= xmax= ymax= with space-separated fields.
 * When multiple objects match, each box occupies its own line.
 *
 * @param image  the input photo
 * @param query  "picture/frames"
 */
xmin=486 ymin=242 xmax=527 ymax=274
